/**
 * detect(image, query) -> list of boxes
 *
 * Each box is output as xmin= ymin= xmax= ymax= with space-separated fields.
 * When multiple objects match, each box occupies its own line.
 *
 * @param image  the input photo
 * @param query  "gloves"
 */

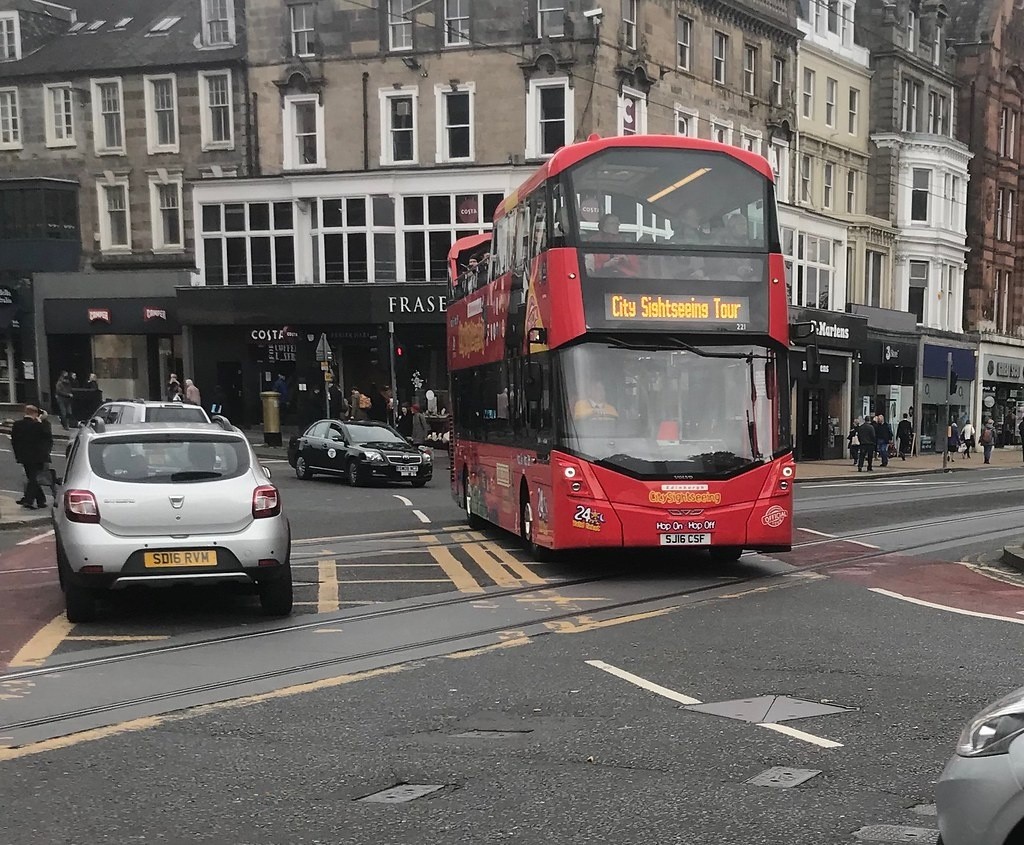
xmin=958 ymin=443 xmax=961 ymax=446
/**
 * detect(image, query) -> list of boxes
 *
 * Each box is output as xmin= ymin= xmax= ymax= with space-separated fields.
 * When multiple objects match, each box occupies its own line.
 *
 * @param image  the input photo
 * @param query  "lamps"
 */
xmin=401 ymin=56 xmax=421 ymax=69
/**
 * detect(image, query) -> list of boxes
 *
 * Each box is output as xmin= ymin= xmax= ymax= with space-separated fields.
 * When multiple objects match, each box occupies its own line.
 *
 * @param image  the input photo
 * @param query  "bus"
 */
xmin=446 ymin=131 xmax=823 ymax=563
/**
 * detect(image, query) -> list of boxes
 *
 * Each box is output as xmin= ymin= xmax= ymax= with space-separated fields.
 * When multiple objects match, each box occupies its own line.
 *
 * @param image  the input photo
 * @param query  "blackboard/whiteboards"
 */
xmin=900 ymin=433 xmax=916 ymax=453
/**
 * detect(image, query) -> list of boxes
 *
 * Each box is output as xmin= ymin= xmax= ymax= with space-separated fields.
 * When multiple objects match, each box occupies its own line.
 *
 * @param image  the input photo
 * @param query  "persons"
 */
xmin=11 ymin=404 xmax=53 ymax=508
xmin=589 ymin=212 xmax=639 ymax=278
xmin=54 ymin=370 xmax=103 ymax=430
xmin=858 ymin=415 xmax=875 ymax=473
xmin=847 ymin=419 xmax=860 ymax=464
xmin=669 ymin=204 xmax=708 ymax=281
xmin=573 ymin=378 xmax=619 ymax=422
xmin=166 ymin=368 xmax=201 ymax=406
xmin=410 ymin=404 xmax=431 ymax=445
xmin=328 ymin=380 xmax=342 ymax=420
xmin=396 ymin=402 xmax=413 ymax=438
xmin=273 ymin=374 xmax=288 ymax=426
xmin=369 ymin=383 xmax=395 ymax=428
xmin=948 ymin=422 xmax=962 ymax=462
xmin=871 ymin=414 xmax=894 ymax=467
xmin=711 ymin=212 xmax=751 ymax=281
xmin=107 ymin=445 xmax=149 ymax=479
xmin=896 ymin=414 xmax=914 ymax=460
xmin=349 ymin=386 xmax=368 ymax=421
xmin=462 ymin=252 xmax=486 ymax=294
xmin=960 ymin=419 xmax=978 ymax=459
xmin=979 ymin=410 xmax=1024 ymax=464
xmin=555 ymin=207 xmax=595 ymax=277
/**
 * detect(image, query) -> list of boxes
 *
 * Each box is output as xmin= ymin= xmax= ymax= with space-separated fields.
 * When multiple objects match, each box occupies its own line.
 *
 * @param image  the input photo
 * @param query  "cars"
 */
xmin=287 ymin=419 xmax=434 ymax=487
xmin=934 ymin=680 xmax=1024 ymax=845
xmin=32 ymin=415 xmax=292 ymax=620
xmin=65 ymin=397 xmax=221 ymax=474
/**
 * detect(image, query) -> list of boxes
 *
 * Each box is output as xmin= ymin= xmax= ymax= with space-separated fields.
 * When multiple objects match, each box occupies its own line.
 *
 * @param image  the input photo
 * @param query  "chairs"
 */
xmin=104 ymin=446 xmax=132 ymax=479
xmin=186 ymin=443 xmax=215 ymax=473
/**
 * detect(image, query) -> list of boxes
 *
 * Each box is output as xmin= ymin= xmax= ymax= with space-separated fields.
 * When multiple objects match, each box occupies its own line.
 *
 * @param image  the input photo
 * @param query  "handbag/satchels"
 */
xmin=852 ymin=435 xmax=860 ymax=445
xmin=970 ymin=435 xmax=975 ymax=447
xmin=442 ymin=431 xmax=450 ymax=444
xmin=888 ymin=443 xmax=897 ymax=458
xmin=958 ymin=442 xmax=967 ymax=453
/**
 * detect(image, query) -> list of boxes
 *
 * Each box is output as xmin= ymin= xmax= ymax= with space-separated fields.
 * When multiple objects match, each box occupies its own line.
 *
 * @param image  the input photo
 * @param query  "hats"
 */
xmin=988 ymin=419 xmax=994 ymax=423
xmin=413 ymin=404 xmax=420 ymax=411
xmin=865 ymin=416 xmax=870 ymax=421
xmin=952 ymin=423 xmax=958 ymax=427
xmin=401 ymin=403 xmax=408 ymax=408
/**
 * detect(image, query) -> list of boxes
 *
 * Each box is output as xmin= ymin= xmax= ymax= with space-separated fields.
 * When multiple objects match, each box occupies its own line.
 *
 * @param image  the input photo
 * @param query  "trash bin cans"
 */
xmin=259 ymin=392 xmax=283 ymax=447
xmin=71 ymin=389 xmax=103 ymax=423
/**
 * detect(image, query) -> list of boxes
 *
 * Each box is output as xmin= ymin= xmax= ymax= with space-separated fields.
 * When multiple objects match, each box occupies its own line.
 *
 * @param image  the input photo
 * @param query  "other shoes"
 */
xmin=854 ymin=460 xmax=858 ymax=465
xmin=38 ymin=503 xmax=47 ymax=507
xmin=857 ymin=467 xmax=862 ymax=471
xmin=880 ymin=463 xmax=887 ymax=466
xmin=25 ymin=505 xmax=37 ymax=510
xmin=16 ymin=499 xmax=23 ymax=504
xmin=867 ymin=467 xmax=872 ymax=471
xmin=902 ymin=454 xmax=905 ymax=460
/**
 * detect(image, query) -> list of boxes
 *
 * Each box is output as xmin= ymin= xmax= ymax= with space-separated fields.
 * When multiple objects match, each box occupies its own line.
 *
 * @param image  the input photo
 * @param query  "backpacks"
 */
xmin=355 ymin=393 xmax=371 ymax=409
xmin=983 ymin=427 xmax=992 ymax=442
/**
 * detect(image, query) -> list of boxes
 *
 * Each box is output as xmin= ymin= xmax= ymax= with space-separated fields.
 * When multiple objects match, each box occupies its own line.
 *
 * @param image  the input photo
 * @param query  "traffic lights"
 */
xmin=396 ymin=345 xmax=405 ymax=358
